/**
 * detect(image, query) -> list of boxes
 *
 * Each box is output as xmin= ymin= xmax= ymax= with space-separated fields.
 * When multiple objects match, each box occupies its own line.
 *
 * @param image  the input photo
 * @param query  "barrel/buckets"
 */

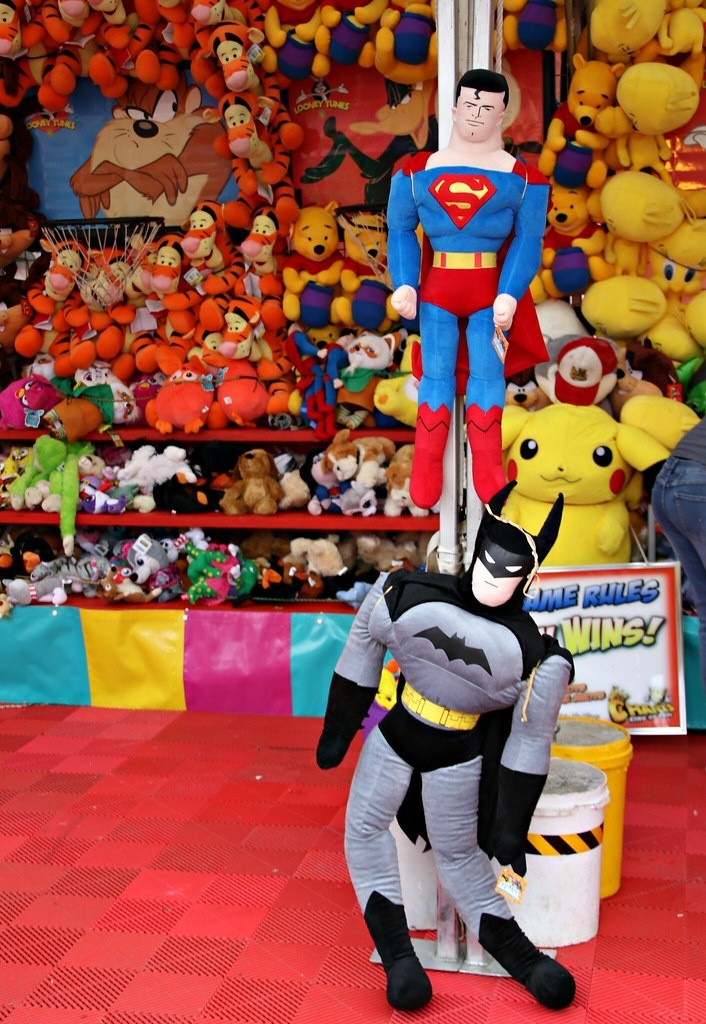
xmin=491 ymin=758 xmax=603 ymax=948
xmin=549 ymin=715 xmax=633 ymax=898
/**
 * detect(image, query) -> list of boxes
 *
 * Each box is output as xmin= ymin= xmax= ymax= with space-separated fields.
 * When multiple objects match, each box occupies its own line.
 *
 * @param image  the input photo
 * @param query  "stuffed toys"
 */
xmin=0 ymin=1 xmax=706 ymax=617
xmin=317 ymin=479 xmax=577 ymax=1009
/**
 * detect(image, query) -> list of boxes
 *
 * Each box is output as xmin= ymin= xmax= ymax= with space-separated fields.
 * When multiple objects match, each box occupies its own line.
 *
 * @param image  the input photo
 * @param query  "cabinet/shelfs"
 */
xmin=0 ymin=419 xmax=438 ymax=713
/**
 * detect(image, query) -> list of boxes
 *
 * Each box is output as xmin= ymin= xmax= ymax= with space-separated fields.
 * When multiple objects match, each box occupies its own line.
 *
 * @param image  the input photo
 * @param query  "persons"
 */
xmin=652 ymin=417 xmax=706 ymax=684
